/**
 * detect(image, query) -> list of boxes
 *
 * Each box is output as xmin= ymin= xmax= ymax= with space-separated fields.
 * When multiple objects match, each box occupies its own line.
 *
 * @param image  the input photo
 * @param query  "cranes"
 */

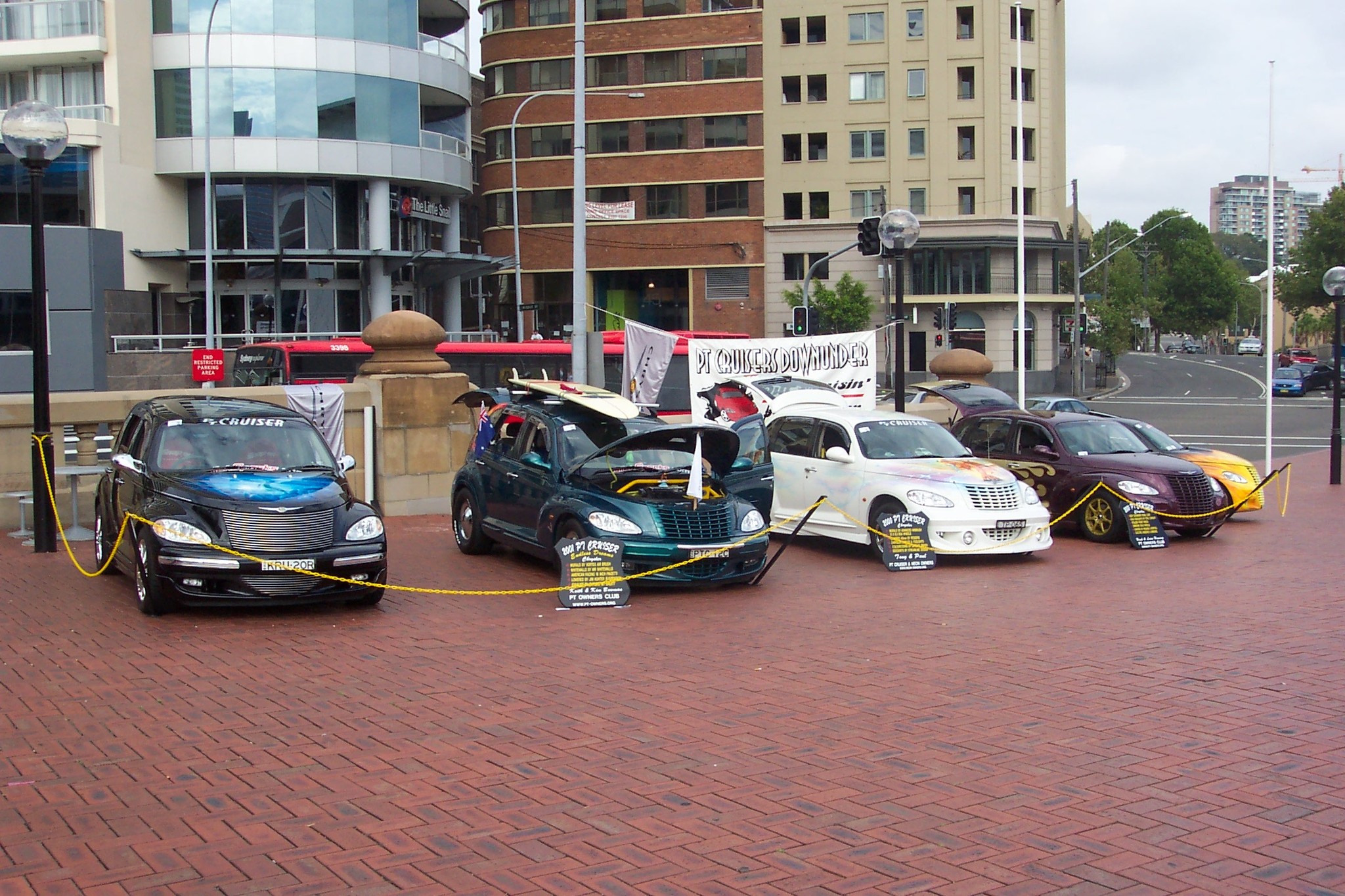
xmin=1276 ymin=152 xmax=1345 ymax=187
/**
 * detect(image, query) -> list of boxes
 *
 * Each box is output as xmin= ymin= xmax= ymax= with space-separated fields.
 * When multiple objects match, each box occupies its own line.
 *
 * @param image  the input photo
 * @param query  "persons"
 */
xmin=494 ymin=329 xmax=501 ymax=342
xmin=484 ymin=324 xmax=493 ymax=342
xmin=531 ymin=329 xmax=543 ymax=340
xmin=1085 ymin=347 xmax=1091 ymax=356
xmin=1209 ymin=338 xmax=1214 ymax=346
xmin=1067 ymin=344 xmax=1071 ymax=359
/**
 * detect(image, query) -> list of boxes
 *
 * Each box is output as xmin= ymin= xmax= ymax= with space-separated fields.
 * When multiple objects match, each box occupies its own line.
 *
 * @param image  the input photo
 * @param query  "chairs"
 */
xmin=497 ymin=423 xmax=524 ymax=457
xmin=533 ymin=428 xmax=551 ymax=463
xmin=1020 ymin=431 xmax=1042 ymax=458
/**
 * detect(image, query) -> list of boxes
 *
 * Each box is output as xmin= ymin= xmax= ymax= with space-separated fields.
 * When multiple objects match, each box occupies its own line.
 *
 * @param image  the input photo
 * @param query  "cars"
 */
xmin=1272 ymin=348 xmax=1345 ymax=398
xmin=93 ymin=395 xmax=389 ymax=615
xmin=448 ymin=386 xmax=775 ymax=588
xmin=1237 ymin=338 xmax=1265 ymax=356
xmin=1167 ymin=339 xmax=1204 ymax=354
xmin=724 ymin=374 xmax=1053 ymax=560
xmin=875 ymin=379 xmax=1265 ymax=543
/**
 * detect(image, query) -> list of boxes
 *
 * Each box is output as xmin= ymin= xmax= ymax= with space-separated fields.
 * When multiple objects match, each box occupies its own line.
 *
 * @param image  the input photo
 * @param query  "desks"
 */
xmin=55 ymin=466 xmax=106 ymax=542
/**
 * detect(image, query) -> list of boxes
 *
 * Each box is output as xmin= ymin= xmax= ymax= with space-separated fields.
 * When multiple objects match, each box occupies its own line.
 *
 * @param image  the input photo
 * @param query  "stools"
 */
xmin=7 ymin=490 xmax=34 ymax=547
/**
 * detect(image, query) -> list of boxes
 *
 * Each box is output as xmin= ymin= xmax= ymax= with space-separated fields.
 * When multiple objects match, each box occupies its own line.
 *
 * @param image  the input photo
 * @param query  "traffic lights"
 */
xmin=1080 ymin=313 xmax=1087 ymax=335
xmin=857 ymin=216 xmax=882 ymax=257
xmin=933 ymin=307 xmax=943 ymax=330
xmin=808 ymin=308 xmax=819 ymax=334
xmin=948 ymin=302 xmax=957 ymax=330
xmin=949 ymin=334 xmax=954 ymax=343
xmin=937 ymin=335 xmax=942 ymax=346
xmin=792 ymin=306 xmax=808 ymax=337
xmin=1222 ymin=338 xmax=1228 ymax=345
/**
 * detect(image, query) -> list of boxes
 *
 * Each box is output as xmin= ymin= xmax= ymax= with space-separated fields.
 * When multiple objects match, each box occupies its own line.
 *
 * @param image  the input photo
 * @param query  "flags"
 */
xmin=687 ymin=434 xmax=702 ymax=502
xmin=621 ymin=319 xmax=680 ymax=403
xmin=474 ymin=401 xmax=495 ymax=457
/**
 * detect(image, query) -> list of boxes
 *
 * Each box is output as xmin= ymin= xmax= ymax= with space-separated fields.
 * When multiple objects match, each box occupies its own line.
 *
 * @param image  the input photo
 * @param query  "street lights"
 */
xmin=877 ymin=209 xmax=920 ymax=413
xmin=511 ymin=91 xmax=646 ymax=342
xmin=1234 ymin=281 xmax=1263 ymax=342
xmin=1242 ymin=257 xmax=1287 ymax=350
xmin=1321 ymin=266 xmax=1345 ymax=484
xmin=1100 ymin=231 xmax=1145 ymax=364
xmin=1073 ymin=212 xmax=1194 ymax=397
xmin=0 ymin=99 xmax=70 ymax=553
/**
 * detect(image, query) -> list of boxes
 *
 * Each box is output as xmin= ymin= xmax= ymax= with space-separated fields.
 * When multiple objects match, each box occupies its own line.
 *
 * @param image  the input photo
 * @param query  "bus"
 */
xmin=230 ymin=329 xmax=752 ymax=424
